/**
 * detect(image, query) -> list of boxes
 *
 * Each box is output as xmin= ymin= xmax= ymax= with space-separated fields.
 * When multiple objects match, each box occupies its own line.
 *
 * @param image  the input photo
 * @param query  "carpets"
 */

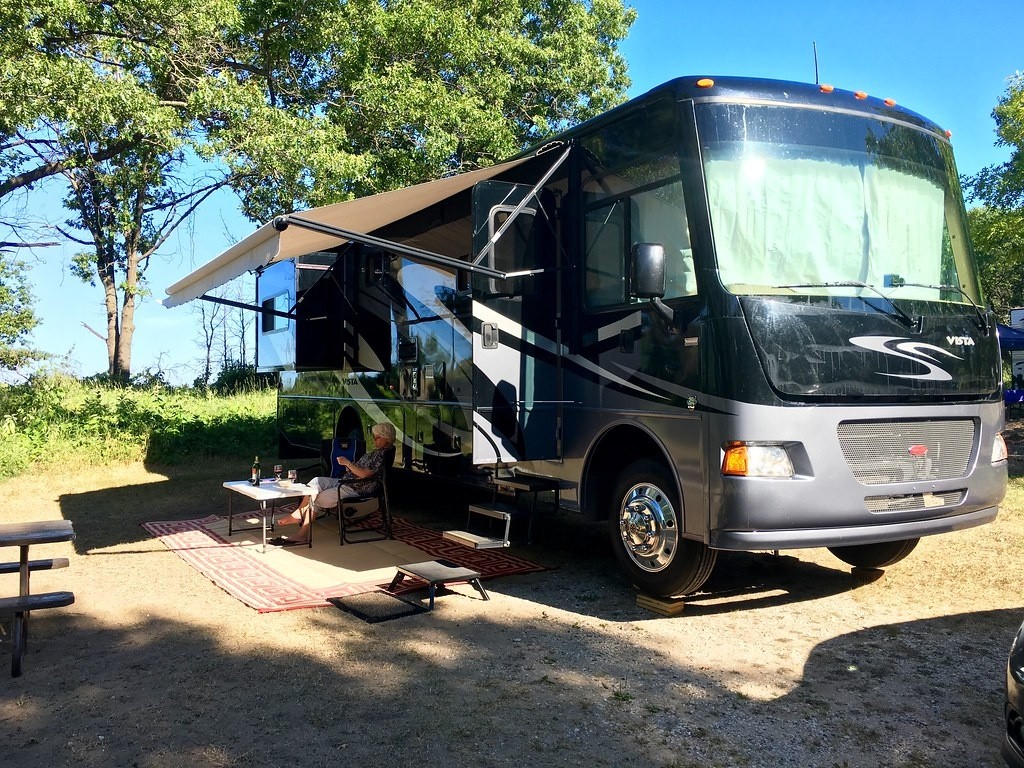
xmin=326 ymin=589 xmax=429 ymax=624
xmin=140 ymin=500 xmax=547 ymax=615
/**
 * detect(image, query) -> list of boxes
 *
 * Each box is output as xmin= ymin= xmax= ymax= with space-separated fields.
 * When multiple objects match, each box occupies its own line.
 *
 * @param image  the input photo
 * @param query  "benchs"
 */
xmin=0 ymin=558 xmax=74 ymax=677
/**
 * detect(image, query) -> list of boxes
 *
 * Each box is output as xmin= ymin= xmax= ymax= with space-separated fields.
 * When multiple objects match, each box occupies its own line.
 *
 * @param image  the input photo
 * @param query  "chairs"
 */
xmin=338 ymin=446 xmax=397 ymax=547
xmin=295 ymin=439 xmax=359 ymax=522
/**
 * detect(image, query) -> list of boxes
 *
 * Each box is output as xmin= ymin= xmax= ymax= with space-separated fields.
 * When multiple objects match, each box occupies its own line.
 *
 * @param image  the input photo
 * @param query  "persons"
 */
xmin=277 ymin=423 xmax=396 ymax=546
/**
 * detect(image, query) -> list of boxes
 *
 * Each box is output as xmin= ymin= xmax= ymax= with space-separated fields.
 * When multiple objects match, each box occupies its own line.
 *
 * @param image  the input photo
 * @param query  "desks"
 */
xmin=223 ymin=478 xmax=318 ymax=554
xmin=1002 ymin=389 xmax=1024 ymax=419
xmin=0 ymin=520 xmax=75 ymax=638
xmin=386 ymin=558 xmax=490 ymax=611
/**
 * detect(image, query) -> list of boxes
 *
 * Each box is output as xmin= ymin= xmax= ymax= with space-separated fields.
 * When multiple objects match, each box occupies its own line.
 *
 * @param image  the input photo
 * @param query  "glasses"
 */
xmin=372 ymin=436 xmax=381 ymax=441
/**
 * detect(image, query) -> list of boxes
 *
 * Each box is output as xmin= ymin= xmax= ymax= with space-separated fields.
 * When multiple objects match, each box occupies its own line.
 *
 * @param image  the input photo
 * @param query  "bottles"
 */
xmin=252 ymin=456 xmax=260 ymax=486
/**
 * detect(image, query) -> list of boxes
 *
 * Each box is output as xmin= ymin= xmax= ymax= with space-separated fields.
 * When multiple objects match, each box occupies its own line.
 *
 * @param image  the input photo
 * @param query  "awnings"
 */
xmin=163 ymin=146 xmax=558 ymax=307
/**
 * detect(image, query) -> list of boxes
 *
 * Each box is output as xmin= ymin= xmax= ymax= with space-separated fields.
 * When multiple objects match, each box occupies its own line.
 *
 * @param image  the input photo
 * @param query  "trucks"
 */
xmin=998 ymin=308 xmax=1024 ymax=406
xmin=162 ymin=77 xmax=1010 ymax=600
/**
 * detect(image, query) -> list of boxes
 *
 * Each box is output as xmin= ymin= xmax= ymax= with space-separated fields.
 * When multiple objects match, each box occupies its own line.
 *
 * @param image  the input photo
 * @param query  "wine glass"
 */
xmin=273 ymin=465 xmax=283 ymax=481
xmin=288 ymin=470 xmax=297 ymax=482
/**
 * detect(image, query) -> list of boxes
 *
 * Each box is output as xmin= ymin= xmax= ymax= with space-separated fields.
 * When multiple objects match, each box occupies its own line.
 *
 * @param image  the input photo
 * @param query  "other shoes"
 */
xmin=269 ymin=537 xmax=298 ymax=545
